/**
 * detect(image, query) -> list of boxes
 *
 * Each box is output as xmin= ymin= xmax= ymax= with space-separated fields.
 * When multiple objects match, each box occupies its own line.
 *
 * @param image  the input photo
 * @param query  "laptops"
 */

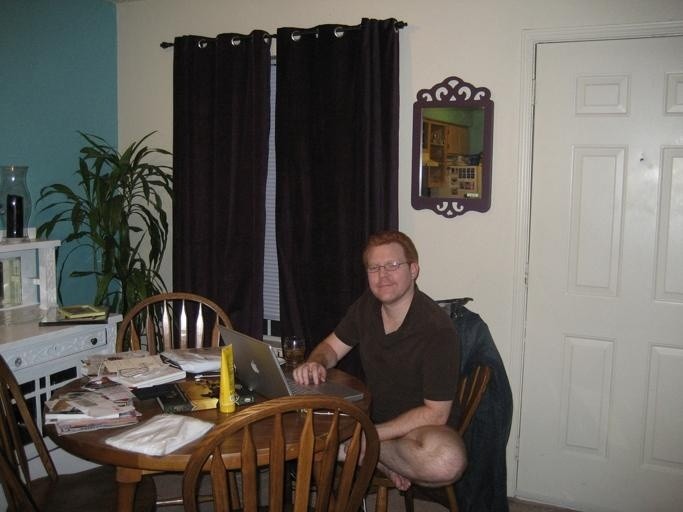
xmin=216 ymin=324 xmax=365 ymax=414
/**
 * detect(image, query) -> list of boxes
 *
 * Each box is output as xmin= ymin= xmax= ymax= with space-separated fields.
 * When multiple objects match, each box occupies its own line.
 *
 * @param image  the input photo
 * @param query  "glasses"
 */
xmin=366 ymin=262 xmax=409 ymax=273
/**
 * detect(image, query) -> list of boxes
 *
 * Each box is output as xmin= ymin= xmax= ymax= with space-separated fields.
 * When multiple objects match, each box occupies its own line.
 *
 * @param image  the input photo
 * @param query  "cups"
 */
xmin=284 ymin=335 xmax=305 ymax=366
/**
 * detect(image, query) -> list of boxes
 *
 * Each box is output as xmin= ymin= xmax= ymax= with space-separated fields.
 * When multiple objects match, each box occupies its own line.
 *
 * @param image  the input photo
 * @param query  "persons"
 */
xmin=288 ymin=227 xmax=470 ymax=491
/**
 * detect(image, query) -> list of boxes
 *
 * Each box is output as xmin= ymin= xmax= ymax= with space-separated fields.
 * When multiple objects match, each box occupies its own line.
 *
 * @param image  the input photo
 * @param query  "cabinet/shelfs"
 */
xmin=0 ymin=226 xmax=125 ymax=464
xmin=422 ymin=116 xmax=482 ymax=194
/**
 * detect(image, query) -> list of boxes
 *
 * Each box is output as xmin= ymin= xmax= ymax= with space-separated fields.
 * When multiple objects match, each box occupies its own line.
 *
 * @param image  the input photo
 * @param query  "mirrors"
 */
xmin=409 ymin=69 xmax=497 ymax=218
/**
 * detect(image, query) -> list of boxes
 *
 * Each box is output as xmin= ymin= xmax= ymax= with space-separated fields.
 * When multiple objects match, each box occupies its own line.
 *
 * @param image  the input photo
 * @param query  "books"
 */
xmin=36 ymin=341 xmax=257 ymax=437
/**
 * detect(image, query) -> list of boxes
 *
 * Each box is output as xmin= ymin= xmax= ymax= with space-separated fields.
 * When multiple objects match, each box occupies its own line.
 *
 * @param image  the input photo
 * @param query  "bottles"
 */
xmin=36 ymin=303 xmax=60 ymax=324
